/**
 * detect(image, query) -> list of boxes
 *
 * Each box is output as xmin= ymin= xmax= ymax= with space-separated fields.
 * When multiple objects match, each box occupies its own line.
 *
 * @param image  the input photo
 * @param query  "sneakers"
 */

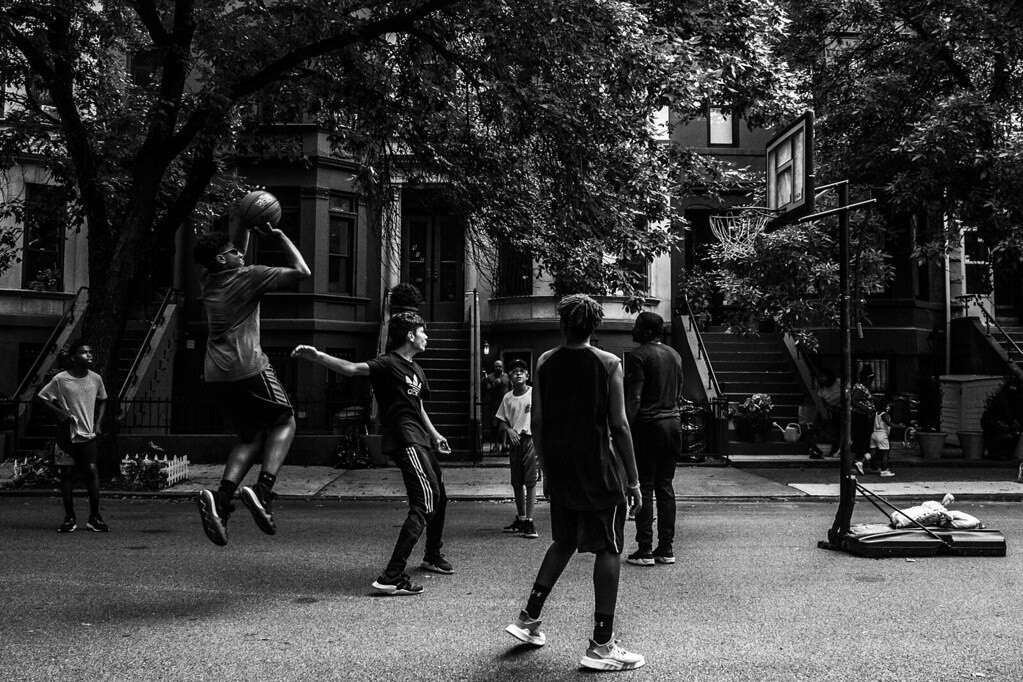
xmin=197 ymin=488 xmax=236 ymax=546
xmin=85 ymin=516 xmax=110 ymax=532
xmin=580 ymin=633 xmax=645 ymax=670
xmin=628 ymin=509 xmax=656 ymax=520
xmin=422 ymin=552 xmax=455 ymax=575
xmin=627 ymin=549 xmax=655 ymax=565
xmin=58 ymin=519 xmax=77 ymax=532
xmin=238 ymin=483 xmax=277 ymax=536
xmin=371 ymin=573 xmax=424 ymax=595
xmin=503 ymin=514 xmax=525 ymax=534
xmin=523 ymin=519 xmax=538 ymax=538
xmin=652 ymin=546 xmax=675 ymax=563
xmin=505 ymin=608 xmax=546 ymax=646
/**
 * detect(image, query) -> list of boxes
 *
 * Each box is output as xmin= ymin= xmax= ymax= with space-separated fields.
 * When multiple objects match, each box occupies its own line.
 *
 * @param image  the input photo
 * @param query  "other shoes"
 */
xmin=880 ymin=468 xmax=895 ymax=477
xmin=501 ymin=446 xmax=510 ymax=453
xmin=855 ymin=461 xmax=864 ymax=475
xmin=489 ymin=446 xmax=500 ymax=452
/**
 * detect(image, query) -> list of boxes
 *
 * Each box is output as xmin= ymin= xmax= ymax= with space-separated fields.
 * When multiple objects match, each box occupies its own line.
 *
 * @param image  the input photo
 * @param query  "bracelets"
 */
xmin=628 ymin=482 xmax=641 ymax=489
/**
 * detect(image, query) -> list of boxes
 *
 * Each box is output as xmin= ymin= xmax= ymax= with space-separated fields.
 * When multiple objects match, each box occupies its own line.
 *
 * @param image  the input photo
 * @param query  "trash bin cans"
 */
xmin=334 ymin=407 xmax=365 ymax=436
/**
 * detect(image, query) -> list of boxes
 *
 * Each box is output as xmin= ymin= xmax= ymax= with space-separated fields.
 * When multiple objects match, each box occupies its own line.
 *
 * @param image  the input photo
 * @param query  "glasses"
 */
xmin=220 ymin=247 xmax=237 ymax=255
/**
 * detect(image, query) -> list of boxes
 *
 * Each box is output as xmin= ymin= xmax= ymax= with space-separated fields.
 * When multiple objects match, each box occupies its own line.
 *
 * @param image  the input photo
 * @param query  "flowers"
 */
xmin=743 ymin=392 xmax=776 ymax=428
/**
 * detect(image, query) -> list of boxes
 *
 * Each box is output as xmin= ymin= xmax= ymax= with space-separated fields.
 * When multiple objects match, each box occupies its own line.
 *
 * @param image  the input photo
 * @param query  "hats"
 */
xmin=510 ymin=359 xmax=528 ymax=371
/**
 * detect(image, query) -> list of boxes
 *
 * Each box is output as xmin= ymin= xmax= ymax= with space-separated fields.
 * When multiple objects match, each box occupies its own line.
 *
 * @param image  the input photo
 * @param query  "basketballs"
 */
xmin=240 ymin=191 xmax=282 ymax=234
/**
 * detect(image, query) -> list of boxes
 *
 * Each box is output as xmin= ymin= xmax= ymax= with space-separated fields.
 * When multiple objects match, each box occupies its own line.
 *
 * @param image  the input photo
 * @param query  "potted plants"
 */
xmin=808 ymin=411 xmax=839 ymax=455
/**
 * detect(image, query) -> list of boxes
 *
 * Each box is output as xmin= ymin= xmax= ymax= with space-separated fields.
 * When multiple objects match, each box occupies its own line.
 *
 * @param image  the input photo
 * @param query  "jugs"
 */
xmin=773 ymin=422 xmax=802 ymax=441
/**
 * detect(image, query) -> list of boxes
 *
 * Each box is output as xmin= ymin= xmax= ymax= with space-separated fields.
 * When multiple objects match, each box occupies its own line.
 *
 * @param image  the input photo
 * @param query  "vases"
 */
xmin=915 ymin=431 xmax=949 ymax=459
xmin=956 ymin=430 xmax=986 ymax=459
xmin=751 ymin=426 xmax=772 ymax=444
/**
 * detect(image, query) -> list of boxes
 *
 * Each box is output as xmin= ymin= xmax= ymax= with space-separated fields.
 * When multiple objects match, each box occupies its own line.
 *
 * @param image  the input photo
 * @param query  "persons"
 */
xmin=37 ymin=339 xmax=111 ymax=532
xmin=817 ymin=364 xmax=880 ymax=473
xmin=291 ymin=312 xmax=455 ymax=593
xmin=190 ymin=221 xmax=310 ymax=547
xmin=625 ymin=312 xmax=681 ymax=565
xmin=854 ymin=400 xmax=906 ymax=477
xmin=482 ymin=361 xmax=513 ymax=453
xmin=505 ymin=294 xmax=644 ymax=669
xmin=495 ymin=360 xmax=540 ymax=537
